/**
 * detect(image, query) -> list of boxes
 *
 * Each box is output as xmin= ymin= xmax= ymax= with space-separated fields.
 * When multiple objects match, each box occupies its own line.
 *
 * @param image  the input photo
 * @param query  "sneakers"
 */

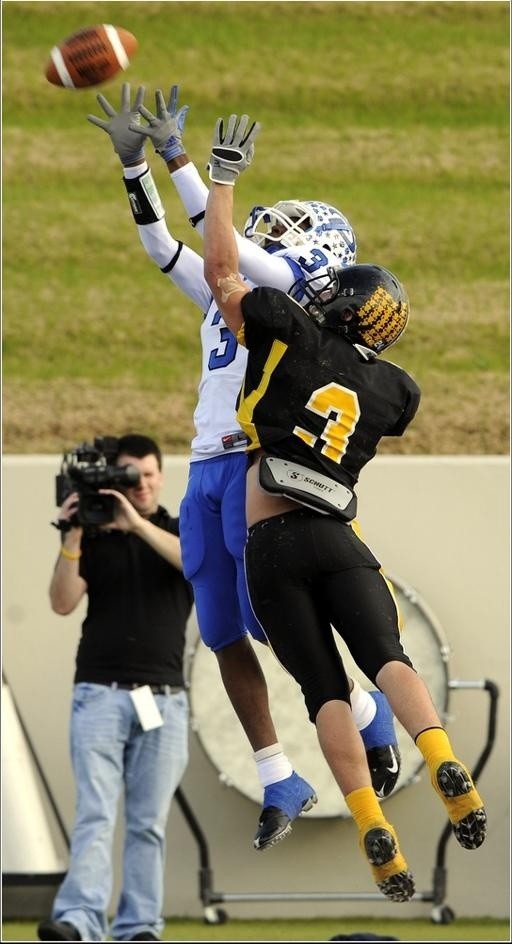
xmin=253 ymin=770 xmax=318 ymax=852
xmin=431 ymin=757 xmax=487 ymax=849
xmin=38 ymin=920 xmax=82 ymax=941
xmin=359 ymin=819 xmax=415 ymax=902
xmin=359 ymin=690 xmax=400 ymax=798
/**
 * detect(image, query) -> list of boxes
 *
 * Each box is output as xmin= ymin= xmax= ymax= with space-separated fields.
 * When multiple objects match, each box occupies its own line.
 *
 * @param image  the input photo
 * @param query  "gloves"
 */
xmin=129 ymin=85 xmax=189 ymax=163
xmin=205 ymin=115 xmax=261 ymax=185
xmin=86 ymin=83 xmax=149 ymax=164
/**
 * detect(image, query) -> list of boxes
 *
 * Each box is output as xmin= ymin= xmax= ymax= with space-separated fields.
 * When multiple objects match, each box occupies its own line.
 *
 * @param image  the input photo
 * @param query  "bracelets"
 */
xmin=60 ymin=547 xmax=82 ymax=561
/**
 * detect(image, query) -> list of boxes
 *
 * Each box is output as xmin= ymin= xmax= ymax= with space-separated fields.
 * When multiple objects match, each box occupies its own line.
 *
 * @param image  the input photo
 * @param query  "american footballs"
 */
xmin=46 ymin=22 xmax=137 ymax=90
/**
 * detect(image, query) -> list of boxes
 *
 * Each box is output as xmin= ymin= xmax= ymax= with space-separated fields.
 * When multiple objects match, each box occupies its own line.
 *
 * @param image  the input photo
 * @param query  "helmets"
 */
xmin=287 ymin=263 xmax=410 ymax=361
xmin=243 ymin=199 xmax=356 ymax=295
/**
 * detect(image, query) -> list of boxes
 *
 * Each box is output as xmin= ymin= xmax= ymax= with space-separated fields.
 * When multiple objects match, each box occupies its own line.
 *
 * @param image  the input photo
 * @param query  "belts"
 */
xmin=102 ymin=682 xmax=184 ymax=694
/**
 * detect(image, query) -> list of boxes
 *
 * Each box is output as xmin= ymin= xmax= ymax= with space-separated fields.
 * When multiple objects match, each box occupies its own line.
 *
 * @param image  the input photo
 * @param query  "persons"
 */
xmin=84 ymin=80 xmax=401 ymax=852
xmin=204 ymin=113 xmax=488 ymax=903
xmin=36 ymin=433 xmax=194 ymax=939
